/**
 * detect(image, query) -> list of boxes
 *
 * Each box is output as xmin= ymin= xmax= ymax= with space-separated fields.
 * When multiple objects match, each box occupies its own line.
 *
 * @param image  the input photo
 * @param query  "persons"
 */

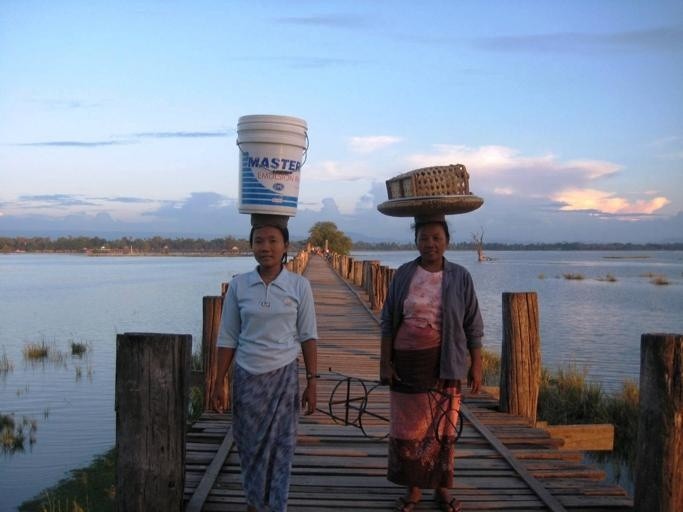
xmin=209 ymin=219 xmax=321 ymax=512
xmin=376 ymin=221 xmax=484 ymax=512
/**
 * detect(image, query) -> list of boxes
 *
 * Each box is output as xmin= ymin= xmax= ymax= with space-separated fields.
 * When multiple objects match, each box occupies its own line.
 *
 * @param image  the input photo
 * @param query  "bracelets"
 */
xmin=305 ymin=373 xmax=319 ymax=380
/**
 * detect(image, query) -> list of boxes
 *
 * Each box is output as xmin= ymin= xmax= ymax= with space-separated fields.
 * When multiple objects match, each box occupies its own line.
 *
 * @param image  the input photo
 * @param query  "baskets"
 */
xmin=376 ymin=164 xmax=485 ymax=215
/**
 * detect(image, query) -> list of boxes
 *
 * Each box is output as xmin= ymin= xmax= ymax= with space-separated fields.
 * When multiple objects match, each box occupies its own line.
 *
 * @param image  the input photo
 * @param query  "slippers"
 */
xmin=395 ymin=495 xmax=419 ymax=512
xmin=437 ymin=497 xmax=461 ymax=512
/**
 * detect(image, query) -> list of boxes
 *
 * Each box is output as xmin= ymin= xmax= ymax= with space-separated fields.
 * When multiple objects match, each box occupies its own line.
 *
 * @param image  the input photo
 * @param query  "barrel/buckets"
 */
xmin=239 ymin=115 xmax=309 ymax=217
